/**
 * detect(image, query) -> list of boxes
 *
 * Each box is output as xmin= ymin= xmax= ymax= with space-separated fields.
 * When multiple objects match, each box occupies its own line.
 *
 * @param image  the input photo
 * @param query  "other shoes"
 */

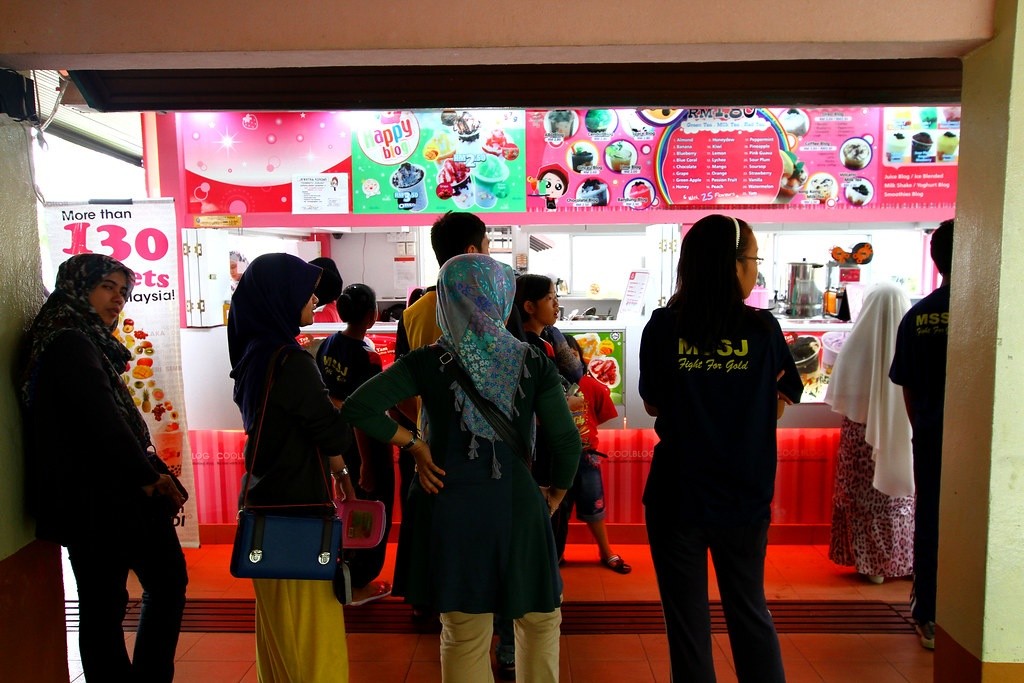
xmin=496 ymin=663 xmax=515 ymax=680
xmin=915 ymin=622 xmax=935 ymax=649
xmin=867 ymin=574 xmax=884 ymax=584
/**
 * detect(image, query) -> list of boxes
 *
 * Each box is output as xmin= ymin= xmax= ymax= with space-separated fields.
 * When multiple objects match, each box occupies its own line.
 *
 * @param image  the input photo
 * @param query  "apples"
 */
xmin=124 ymin=319 xmax=134 ymax=326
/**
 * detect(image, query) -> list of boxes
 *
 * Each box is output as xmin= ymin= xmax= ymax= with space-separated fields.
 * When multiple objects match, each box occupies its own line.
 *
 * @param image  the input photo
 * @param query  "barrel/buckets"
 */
xmin=596 ymin=315 xmax=613 ymax=320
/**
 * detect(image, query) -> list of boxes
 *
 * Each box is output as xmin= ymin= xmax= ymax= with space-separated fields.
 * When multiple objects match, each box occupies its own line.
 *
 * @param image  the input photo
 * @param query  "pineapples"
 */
xmin=142 ymin=389 xmax=151 ymax=413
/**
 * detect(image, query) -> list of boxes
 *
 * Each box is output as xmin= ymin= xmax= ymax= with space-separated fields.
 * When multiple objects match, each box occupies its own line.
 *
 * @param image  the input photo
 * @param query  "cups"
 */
xmin=580 ymin=185 xmax=607 ymax=206
xmin=610 ymin=151 xmax=632 ymax=172
xmin=572 ymin=154 xmax=593 ymax=173
xmin=471 ymin=160 xmax=508 ymax=209
xmin=434 ymin=148 xmax=458 ymax=170
xmin=936 ymin=136 xmax=959 ymax=161
xmin=818 ymin=332 xmax=850 ymax=384
xmin=630 ymin=189 xmax=651 ymax=209
xmin=911 ymin=137 xmax=933 ymax=163
xmin=436 ymin=167 xmax=475 ymax=209
xmin=539 ymin=181 xmax=546 ymax=195
xmin=152 ymin=431 xmax=184 ymax=477
xmin=389 ymin=166 xmax=428 ymax=213
xmin=778 ymin=173 xmax=808 ymax=204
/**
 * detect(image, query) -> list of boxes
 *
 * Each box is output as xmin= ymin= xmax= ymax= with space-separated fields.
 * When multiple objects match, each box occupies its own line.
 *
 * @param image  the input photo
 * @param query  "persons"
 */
xmin=227 ymin=209 xmax=633 ymax=683
xmin=229 ymin=250 xmax=249 ymax=296
xmin=823 ymin=285 xmax=916 ymax=583
xmin=639 ymin=215 xmax=804 ymax=682
xmin=12 ymin=254 xmax=190 ymax=683
xmin=308 ymin=258 xmax=343 ymax=323
xmin=888 ymin=218 xmax=954 ymax=649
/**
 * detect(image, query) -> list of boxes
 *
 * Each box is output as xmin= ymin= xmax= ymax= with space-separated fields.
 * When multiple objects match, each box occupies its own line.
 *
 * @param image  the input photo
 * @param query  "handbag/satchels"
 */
xmin=333 ymin=562 xmax=354 ymax=604
xmin=228 ymin=507 xmax=342 ymax=581
xmin=145 ymin=452 xmax=188 ymax=515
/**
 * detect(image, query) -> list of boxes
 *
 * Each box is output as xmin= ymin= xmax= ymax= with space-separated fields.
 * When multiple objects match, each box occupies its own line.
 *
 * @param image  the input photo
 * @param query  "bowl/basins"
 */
xmin=793 ymin=335 xmax=821 ymax=373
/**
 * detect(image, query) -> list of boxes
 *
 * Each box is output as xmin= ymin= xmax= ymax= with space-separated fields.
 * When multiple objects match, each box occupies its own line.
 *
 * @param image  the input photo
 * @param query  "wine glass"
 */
xmin=530 ymin=179 xmax=537 ymax=195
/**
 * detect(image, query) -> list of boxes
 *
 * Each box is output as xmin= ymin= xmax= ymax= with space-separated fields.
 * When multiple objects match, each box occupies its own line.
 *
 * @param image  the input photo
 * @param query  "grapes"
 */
xmin=152 ymin=404 xmax=165 ymax=421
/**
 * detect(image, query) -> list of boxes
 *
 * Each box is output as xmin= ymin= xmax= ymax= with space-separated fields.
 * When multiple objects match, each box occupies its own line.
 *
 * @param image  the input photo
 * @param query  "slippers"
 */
xmin=348 ymin=581 xmax=392 ymax=606
xmin=601 ymin=553 xmax=632 ymax=574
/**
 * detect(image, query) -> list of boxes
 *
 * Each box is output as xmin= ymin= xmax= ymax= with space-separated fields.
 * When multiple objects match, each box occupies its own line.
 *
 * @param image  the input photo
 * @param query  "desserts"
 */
xmin=438 ymin=159 xmax=471 ymax=196
xmin=574 ymin=333 xmax=600 ymax=359
xmin=392 ymin=162 xmax=423 ymax=188
xmin=590 ymin=357 xmax=619 ymax=386
xmin=425 ymin=130 xmax=454 ymax=157
xmin=788 ymin=337 xmax=819 ymax=373
xmin=482 ymin=129 xmax=507 ymax=156
xmin=458 ymin=112 xmax=480 ymax=141
xmin=822 ymin=332 xmax=848 ymax=365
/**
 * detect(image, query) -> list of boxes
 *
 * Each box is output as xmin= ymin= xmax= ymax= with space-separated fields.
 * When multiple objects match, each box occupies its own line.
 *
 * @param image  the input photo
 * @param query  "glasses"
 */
xmin=739 ymin=255 xmax=764 ymax=265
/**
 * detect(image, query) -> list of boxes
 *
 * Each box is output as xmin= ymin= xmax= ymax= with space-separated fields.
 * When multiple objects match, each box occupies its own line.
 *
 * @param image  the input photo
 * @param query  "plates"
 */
xmin=589 ymin=357 xmax=621 ymax=389
xmin=805 ymin=174 xmax=838 ymax=204
xmin=840 ymin=137 xmax=872 ymax=170
xmin=843 ymin=176 xmax=875 ymax=206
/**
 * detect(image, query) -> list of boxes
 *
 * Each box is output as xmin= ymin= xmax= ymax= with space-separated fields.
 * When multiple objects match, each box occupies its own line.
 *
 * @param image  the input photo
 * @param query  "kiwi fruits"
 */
xmin=134 ymin=347 xmax=143 ymax=355
xmin=145 ymin=348 xmax=155 ymax=356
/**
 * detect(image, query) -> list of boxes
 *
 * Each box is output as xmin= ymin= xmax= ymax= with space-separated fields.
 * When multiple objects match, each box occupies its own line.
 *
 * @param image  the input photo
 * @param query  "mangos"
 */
xmin=132 ymin=365 xmax=153 ymax=379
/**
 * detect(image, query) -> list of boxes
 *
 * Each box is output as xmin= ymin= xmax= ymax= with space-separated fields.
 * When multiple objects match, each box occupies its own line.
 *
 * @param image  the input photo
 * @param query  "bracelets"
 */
xmin=331 ymin=465 xmax=349 ymax=479
xmin=398 ymin=430 xmax=417 ymax=449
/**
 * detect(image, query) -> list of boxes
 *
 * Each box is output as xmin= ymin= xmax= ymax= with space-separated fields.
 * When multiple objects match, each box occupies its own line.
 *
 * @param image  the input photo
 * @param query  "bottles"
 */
xmin=223 ymin=301 xmax=230 ymax=325
